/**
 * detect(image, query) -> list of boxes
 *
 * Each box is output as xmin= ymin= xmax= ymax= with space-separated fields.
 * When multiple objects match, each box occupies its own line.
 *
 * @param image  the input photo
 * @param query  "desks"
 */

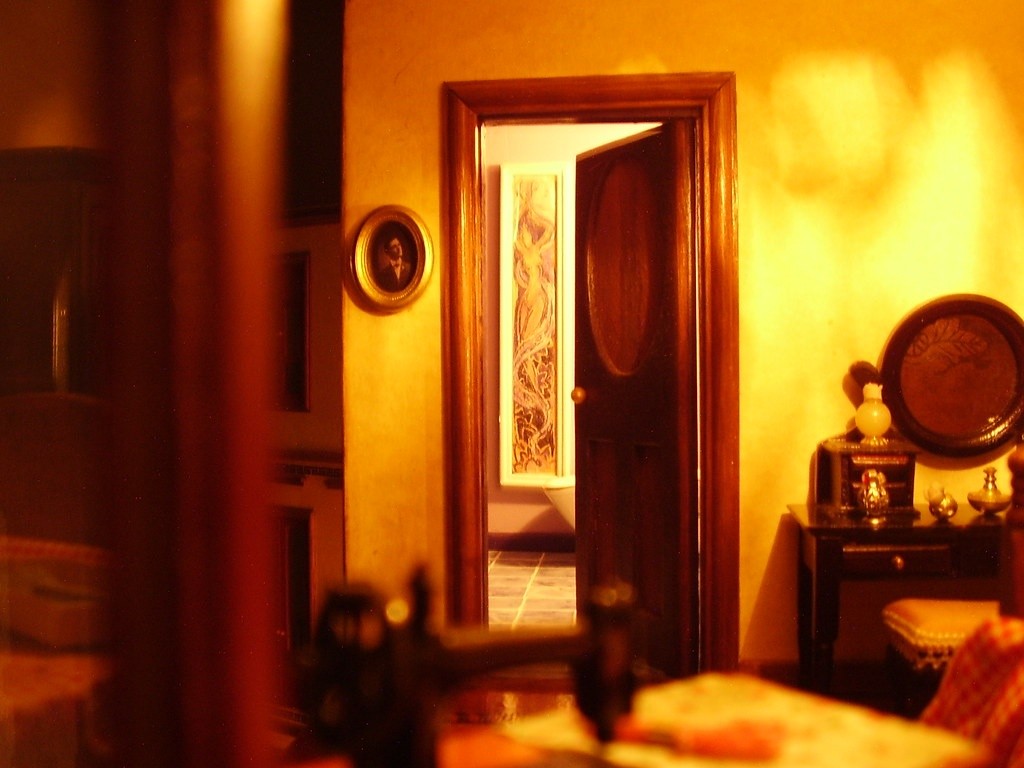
xmin=785 ymin=502 xmax=1011 ymax=695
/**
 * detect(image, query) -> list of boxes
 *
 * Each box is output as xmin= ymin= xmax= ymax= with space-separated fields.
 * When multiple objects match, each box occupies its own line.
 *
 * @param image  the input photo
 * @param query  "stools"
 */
xmin=882 ymin=600 xmax=1000 ymax=723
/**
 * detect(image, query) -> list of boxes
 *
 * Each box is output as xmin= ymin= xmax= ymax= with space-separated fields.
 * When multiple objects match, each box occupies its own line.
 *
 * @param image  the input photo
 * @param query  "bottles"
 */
xmin=854 ymin=382 xmax=893 ymax=447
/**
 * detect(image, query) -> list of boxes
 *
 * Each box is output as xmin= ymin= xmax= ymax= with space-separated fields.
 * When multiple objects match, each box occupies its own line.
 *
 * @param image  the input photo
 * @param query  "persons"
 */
xmin=378 ymin=236 xmax=415 ymax=292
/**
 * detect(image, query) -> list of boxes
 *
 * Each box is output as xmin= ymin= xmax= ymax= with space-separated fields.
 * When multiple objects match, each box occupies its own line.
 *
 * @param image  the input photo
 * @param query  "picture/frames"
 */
xmin=349 ymin=204 xmax=434 ymax=309
